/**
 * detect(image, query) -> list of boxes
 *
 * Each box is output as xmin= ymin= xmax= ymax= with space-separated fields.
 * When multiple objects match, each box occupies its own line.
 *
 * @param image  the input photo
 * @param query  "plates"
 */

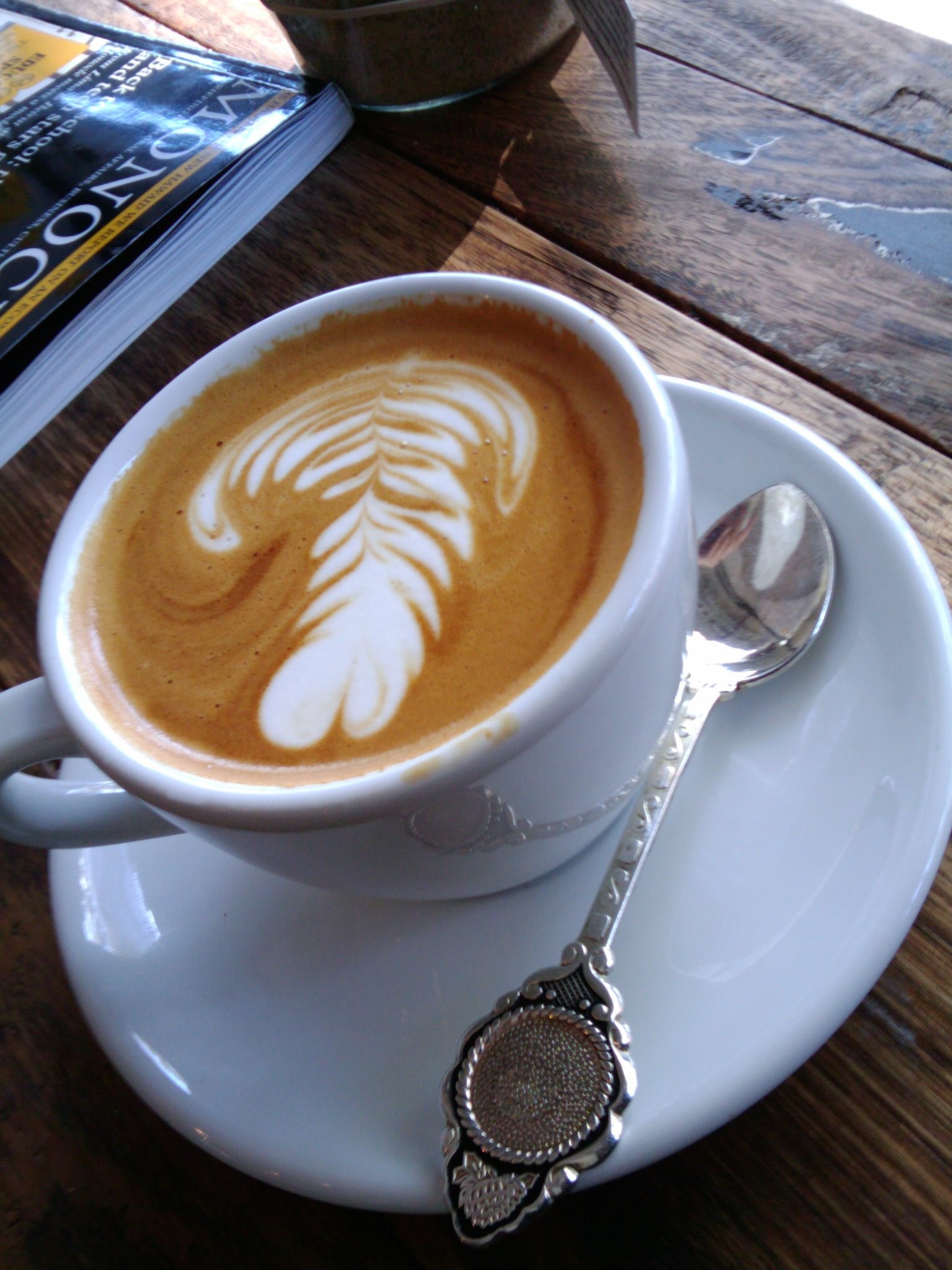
xmin=50 ymin=374 xmax=952 ymax=1213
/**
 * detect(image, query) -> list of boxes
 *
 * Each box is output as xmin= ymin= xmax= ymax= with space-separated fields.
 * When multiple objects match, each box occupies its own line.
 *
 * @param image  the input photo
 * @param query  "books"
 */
xmin=1 ymin=0 xmax=355 ymax=465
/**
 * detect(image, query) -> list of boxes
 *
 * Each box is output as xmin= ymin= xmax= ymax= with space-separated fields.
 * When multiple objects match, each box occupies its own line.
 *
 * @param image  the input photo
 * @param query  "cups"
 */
xmin=262 ymin=0 xmax=583 ymax=112
xmin=7 ymin=272 xmax=691 ymax=899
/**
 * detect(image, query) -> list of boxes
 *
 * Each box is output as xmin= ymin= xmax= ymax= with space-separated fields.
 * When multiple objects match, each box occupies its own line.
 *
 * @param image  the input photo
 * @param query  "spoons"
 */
xmin=442 ymin=480 xmax=836 ymax=1250
